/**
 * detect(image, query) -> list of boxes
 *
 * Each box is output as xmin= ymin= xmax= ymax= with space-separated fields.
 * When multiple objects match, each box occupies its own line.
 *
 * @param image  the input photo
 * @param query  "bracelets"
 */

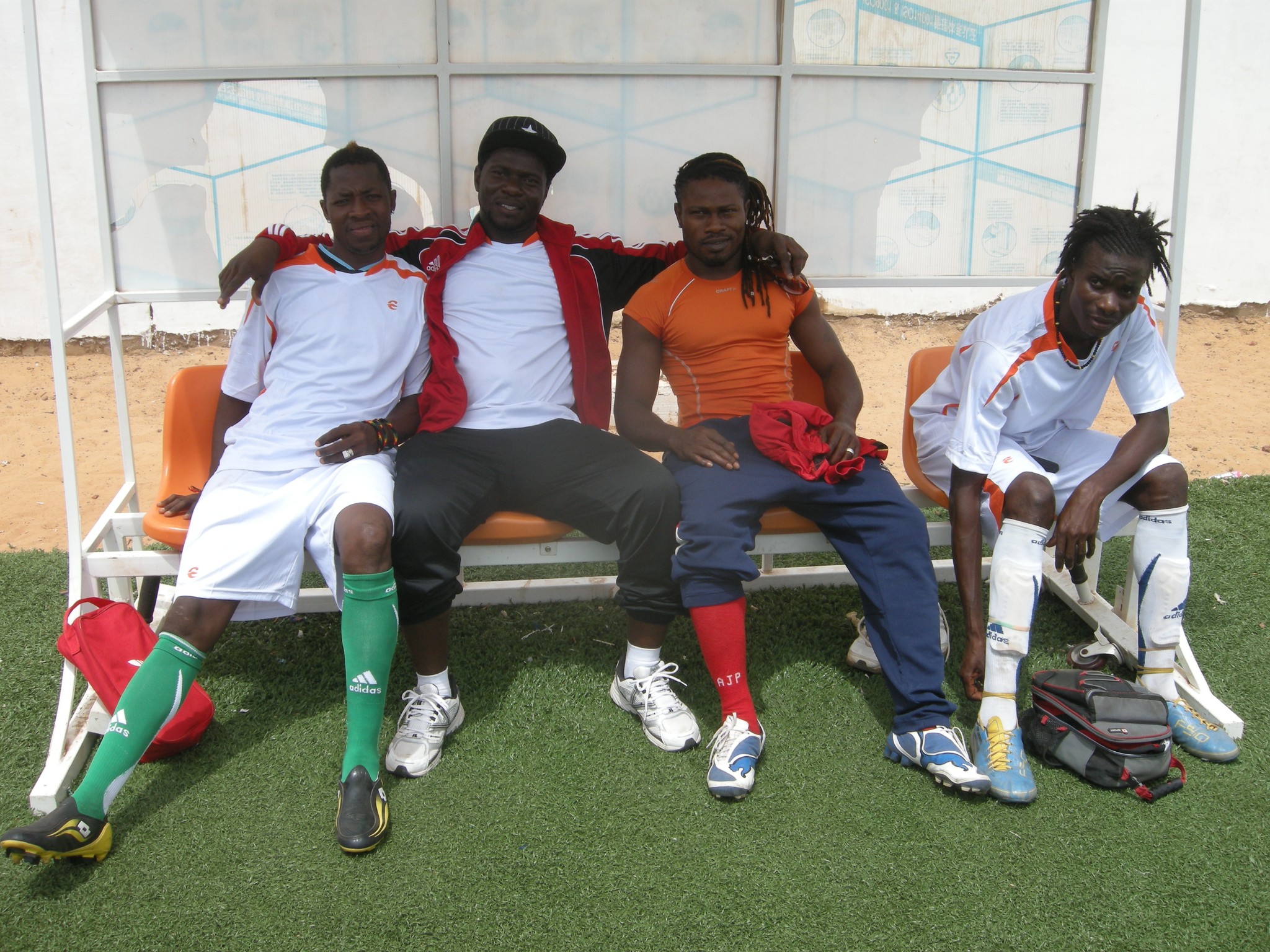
xmin=361 ymin=418 xmax=398 ymax=454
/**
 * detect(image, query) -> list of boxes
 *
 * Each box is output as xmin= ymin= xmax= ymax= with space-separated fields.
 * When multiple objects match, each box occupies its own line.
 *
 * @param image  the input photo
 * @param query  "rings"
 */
xmin=342 ymin=448 xmax=354 ymax=460
xmin=846 ymin=448 xmax=855 ymax=457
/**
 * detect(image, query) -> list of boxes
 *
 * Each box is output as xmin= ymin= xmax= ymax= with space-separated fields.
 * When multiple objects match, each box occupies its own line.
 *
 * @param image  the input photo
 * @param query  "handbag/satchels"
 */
xmin=58 ymin=596 xmax=215 ymax=762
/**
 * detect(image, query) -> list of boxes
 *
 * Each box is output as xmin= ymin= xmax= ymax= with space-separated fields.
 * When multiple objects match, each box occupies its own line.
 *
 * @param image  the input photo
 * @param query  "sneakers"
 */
xmin=971 ymin=691 xmax=1037 ymax=802
xmin=1136 ymin=647 xmax=1238 ymax=762
xmin=705 ymin=712 xmax=766 ymax=798
xmin=883 ymin=724 xmax=991 ymax=794
xmin=335 ymin=765 xmax=389 ymax=853
xmin=608 ymin=650 xmax=701 ymax=751
xmin=0 ymin=795 xmax=112 ymax=862
xmin=386 ymin=674 xmax=465 ymax=777
xmin=847 ymin=602 xmax=950 ymax=673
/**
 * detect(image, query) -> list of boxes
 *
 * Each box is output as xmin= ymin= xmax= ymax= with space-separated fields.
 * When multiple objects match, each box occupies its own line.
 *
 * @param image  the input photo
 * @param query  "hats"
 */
xmin=478 ymin=117 xmax=567 ymax=180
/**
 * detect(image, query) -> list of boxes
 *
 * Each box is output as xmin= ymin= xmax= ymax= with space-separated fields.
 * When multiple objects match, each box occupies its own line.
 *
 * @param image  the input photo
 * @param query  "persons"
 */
xmin=614 ymin=152 xmax=992 ymax=802
xmin=218 ymin=116 xmax=806 ymax=779
xmin=0 ymin=139 xmax=436 ymax=868
xmin=909 ymin=192 xmax=1240 ymax=804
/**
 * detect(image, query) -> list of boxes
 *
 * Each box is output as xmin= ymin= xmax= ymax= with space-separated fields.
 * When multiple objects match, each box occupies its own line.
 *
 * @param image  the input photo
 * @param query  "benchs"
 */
xmin=132 ymin=349 xmax=1096 ymax=680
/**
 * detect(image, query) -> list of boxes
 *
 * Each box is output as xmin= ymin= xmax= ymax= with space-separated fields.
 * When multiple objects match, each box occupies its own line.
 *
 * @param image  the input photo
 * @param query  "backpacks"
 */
xmin=1019 ymin=669 xmax=1186 ymax=803
